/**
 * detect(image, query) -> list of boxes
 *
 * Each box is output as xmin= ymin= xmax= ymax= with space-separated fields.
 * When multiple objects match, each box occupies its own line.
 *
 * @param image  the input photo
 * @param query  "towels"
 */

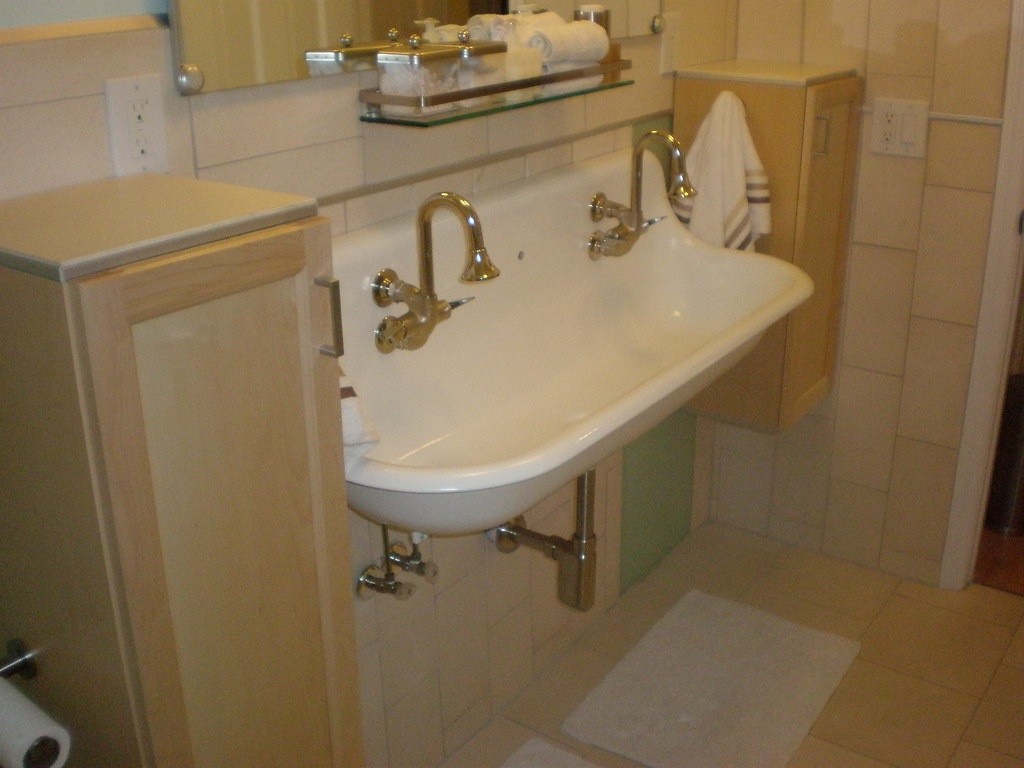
xmin=669 ymin=90 xmax=772 ymax=256
xmin=436 ymin=24 xmax=465 ymax=45
xmin=467 ymin=11 xmax=611 ymax=99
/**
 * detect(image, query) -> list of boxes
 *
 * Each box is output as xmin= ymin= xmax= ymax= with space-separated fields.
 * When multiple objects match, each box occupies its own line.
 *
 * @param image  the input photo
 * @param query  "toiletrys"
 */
xmin=415 ymin=17 xmax=441 ymax=48
xmin=511 ymin=3 xmax=541 ymax=15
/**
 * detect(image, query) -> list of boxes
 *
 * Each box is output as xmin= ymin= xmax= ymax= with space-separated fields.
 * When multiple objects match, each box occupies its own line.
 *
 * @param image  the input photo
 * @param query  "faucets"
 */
xmin=587 ymin=129 xmax=697 ymax=263
xmin=372 ymin=191 xmax=501 ymax=354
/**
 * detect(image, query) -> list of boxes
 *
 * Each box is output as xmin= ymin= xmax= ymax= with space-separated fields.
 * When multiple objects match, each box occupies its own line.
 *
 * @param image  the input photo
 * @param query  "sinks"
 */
xmin=333 ymin=150 xmax=816 ymax=540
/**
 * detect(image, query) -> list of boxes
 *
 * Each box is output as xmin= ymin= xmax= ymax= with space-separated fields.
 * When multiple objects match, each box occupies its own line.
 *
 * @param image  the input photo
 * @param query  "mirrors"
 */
xmin=171 ymin=0 xmax=514 ymax=98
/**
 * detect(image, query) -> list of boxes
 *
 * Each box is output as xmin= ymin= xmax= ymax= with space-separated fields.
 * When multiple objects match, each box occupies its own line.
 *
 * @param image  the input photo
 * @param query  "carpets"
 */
xmin=562 ymin=590 xmax=862 ymax=768
xmin=501 ymin=738 xmax=601 ymax=768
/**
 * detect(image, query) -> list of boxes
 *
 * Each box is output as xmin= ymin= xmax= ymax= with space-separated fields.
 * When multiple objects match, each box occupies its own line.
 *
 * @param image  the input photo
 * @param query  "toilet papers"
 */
xmin=0 ymin=673 xmax=71 ymax=767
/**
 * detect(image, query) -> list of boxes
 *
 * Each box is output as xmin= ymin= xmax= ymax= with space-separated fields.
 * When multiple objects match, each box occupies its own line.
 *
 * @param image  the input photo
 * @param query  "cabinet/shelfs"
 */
xmin=0 ymin=174 xmax=363 ymax=767
xmin=671 ymin=57 xmax=866 ymax=432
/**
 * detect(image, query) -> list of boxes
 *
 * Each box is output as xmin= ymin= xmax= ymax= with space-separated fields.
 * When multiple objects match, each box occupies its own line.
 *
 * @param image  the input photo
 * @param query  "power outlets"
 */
xmin=869 ymin=97 xmax=928 ymax=158
xmin=106 ymin=74 xmax=173 ymax=176
